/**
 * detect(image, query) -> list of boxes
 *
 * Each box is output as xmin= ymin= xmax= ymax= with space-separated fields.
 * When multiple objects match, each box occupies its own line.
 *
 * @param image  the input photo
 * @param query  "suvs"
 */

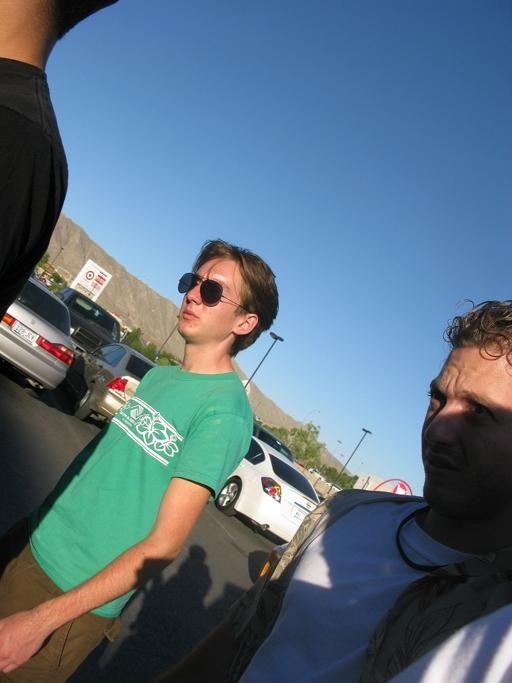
xmin=52 ymin=286 xmax=124 ymax=363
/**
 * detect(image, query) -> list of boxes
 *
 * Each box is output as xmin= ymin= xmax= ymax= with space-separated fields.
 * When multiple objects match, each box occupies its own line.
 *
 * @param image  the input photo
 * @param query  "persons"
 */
xmin=0 ymin=2 xmax=123 ymax=327
xmin=148 ymin=300 xmax=512 ymax=682
xmin=1 ymin=239 xmax=281 ymax=681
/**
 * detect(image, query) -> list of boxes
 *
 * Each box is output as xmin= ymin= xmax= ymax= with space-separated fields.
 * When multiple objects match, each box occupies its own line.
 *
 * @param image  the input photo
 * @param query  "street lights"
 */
xmin=324 ymin=428 xmax=373 ymax=499
xmin=241 ymin=332 xmax=284 ymax=388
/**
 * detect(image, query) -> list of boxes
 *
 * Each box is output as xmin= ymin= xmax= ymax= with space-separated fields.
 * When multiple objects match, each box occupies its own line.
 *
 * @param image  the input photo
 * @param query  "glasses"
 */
xmin=178 ymin=270 xmax=246 ymax=314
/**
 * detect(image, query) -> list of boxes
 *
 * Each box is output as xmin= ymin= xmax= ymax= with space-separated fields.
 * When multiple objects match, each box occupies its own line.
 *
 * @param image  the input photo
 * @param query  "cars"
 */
xmin=61 ymin=341 xmax=157 ymax=427
xmin=214 ymin=422 xmax=320 ymax=544
xmin=0 ymin=275 xmax=75 ymax=401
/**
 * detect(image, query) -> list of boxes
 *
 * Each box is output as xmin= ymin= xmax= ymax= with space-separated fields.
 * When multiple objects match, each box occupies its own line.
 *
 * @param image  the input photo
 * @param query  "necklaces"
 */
xmin=396 ymin=506 xmax=453 ymax=571
